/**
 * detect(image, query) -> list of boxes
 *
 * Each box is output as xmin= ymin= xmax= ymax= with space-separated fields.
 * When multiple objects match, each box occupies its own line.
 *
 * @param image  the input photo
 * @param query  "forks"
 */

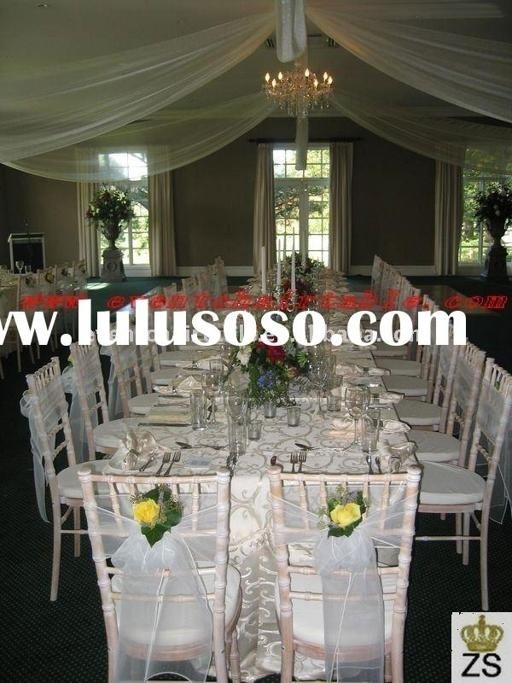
xmin=163 ymin=451 xmax=182 ymax=476
xmin=296 ymin=452 xmax=306 ymax=472
xmin=290 ymin=451 xmax=298 ymax=473
xmin=156 ymin=450 xmax=172 ymax=476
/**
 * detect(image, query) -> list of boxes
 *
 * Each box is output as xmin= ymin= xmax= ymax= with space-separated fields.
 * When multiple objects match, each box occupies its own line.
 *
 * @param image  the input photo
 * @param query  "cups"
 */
xmin=25 ymin=265 xmax=32 ymax=273
xmin=261 ymin=262 xmax=380 ymax=453
xmin=188 ymin=351 xmax=262 ymax=459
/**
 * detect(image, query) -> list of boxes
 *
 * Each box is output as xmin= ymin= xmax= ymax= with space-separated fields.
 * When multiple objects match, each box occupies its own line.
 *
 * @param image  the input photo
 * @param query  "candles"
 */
xmin=258 ymin=225 xmax=311 ymax=294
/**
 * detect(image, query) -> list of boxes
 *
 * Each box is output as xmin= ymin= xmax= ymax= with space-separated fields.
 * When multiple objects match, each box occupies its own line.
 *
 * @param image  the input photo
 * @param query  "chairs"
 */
xmin=0 ymin=254 xmax=512 ymax=682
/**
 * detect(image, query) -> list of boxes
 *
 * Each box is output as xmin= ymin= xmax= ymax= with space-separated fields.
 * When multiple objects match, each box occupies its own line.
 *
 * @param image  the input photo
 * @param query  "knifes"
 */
xmin=374 ymin=456 xmax=382 ymax=473
xmin=366 ymin=455 xmax=375 ymax=474
xmin=153 ymin=401 xmax=182 ymax=407
xmin=137 ymin=421 xmax=190 ymax=426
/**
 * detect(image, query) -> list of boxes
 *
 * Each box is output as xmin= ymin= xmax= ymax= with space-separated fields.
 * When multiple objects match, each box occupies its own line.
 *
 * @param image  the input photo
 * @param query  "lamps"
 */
xmin=261 ymin=60 xmax=334 ymax=119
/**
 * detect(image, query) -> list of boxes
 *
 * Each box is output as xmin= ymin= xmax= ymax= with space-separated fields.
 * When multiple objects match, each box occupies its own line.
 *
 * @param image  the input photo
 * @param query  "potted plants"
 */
xmin=469 ymin=179 xmax=512 ymax=241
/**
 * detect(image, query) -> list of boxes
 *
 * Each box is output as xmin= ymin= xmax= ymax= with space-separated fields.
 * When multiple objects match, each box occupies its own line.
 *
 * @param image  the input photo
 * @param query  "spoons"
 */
xmin=175 ymin=440 xmax=219 ymax=449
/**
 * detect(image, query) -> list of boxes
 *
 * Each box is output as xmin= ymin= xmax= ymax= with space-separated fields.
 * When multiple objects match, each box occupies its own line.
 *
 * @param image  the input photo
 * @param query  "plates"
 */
xmin=381 ymin=418 xmax=402 ymax=433
xmin=391 ymin=442 xmax=409 ymax=450
xmin=153 ymin=384 xmax=176 ymax=395
xmin=380 ymin=392 xmax=401 ymax=403
xmin=108 ymin=454 xmax=151 ymax=472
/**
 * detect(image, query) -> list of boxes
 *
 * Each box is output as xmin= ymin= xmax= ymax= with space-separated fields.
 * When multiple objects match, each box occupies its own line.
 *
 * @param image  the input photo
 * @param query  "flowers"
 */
xmin=81 ymin=183 xmax=138 ymax=247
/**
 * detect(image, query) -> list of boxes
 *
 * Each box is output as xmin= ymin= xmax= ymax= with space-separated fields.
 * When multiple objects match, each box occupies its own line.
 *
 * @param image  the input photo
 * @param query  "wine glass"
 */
xmin=16 ymin=259 xmax=25 ymax=273
